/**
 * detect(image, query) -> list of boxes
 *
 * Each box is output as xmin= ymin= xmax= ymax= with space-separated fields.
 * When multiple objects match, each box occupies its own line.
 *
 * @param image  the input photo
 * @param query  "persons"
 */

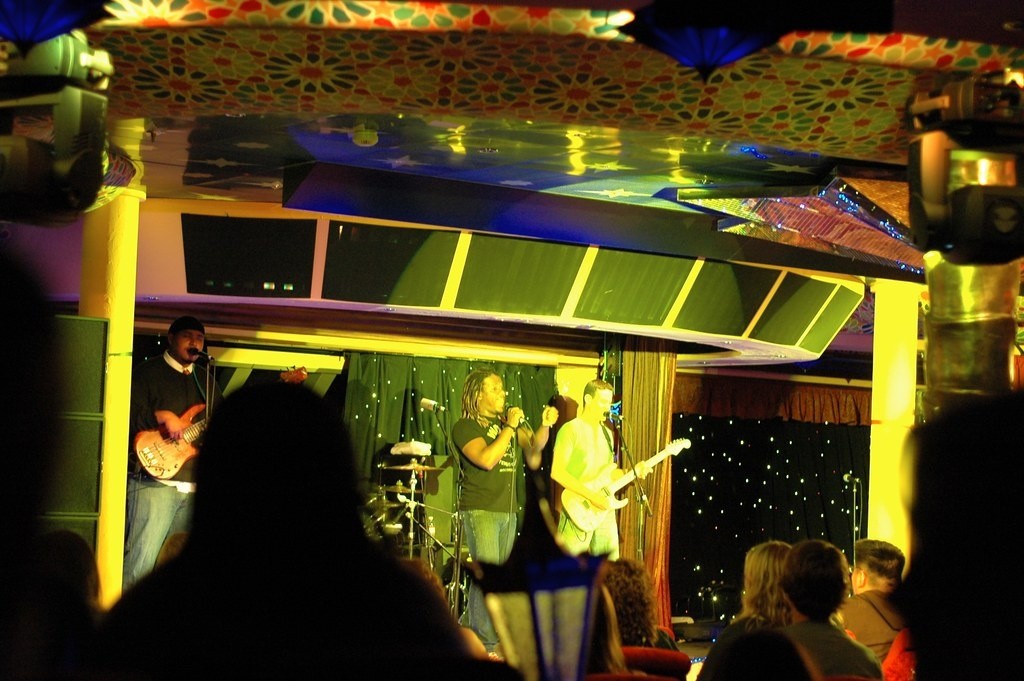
xmin=598 ymin=558 xmax=680 ymax=652
xmin=122 ymin=316 xmax=224 ymax=596
xmin=452 ymin=369 xmax=559 ymax=661
xmin=884 ymin=388 xmax=1024 ymax=680
xmin=82 ymin=381 xmax=526 ymax=681
xmin=550 ymin=380 xmax=653 ymax=561
xmin=696 ymin=540 xmax=905 ymax=681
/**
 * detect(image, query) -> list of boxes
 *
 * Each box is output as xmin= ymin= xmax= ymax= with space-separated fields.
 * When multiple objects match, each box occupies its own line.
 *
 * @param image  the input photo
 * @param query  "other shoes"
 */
xmin=487 ymin=651 xmax=503 ymax=661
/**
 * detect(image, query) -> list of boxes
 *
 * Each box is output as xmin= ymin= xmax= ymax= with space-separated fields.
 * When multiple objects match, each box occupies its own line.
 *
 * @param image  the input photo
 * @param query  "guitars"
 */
xmin=560 ymin=437 xmax=693 ymax=534
xmin=134 ymin=365 xmax=308 ymax=480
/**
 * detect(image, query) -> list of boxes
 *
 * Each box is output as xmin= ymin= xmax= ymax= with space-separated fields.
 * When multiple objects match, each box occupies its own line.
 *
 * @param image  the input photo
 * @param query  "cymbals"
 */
xmin=379 ymin=484 xmax=424 ymax=494
xmin=366 ymin=498 xmax=403 ymax=509
xmin=384 ymin=464 xmax=447 ymax=473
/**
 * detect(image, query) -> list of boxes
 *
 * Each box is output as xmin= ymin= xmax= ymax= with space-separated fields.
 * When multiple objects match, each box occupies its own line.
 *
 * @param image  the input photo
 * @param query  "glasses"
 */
xmin=849 ymin=565 xmax=866 ymax=574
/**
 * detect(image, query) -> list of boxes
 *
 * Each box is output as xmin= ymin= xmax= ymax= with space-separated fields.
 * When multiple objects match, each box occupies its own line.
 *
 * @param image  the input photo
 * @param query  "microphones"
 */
xmin=843 ymin=473 xmax=861 ymax=482
xmin=505 ymin=402 xmax=526 ymax=426
xmin=187 ymin=347 xmax=214 ymax=360
xmin=420 ymin=398 xmax=449 ymax=412
xmin=603 ymin=412 xmax=626 ymax=420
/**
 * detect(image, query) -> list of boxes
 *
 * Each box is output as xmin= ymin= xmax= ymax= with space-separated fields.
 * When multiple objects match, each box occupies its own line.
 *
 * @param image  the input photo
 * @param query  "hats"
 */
xmin=167 ymin=316 xmax=205 ymax=336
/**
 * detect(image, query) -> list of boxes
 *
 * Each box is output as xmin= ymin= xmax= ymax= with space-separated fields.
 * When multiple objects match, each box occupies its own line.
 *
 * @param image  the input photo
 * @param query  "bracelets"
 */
xmin=502 ymin=423 xmax=516 ymax=432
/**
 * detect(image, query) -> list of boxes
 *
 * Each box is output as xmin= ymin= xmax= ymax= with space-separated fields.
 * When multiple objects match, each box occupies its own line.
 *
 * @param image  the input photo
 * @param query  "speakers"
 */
xmin=36 ymin=312 xmax=110 ymax=561
